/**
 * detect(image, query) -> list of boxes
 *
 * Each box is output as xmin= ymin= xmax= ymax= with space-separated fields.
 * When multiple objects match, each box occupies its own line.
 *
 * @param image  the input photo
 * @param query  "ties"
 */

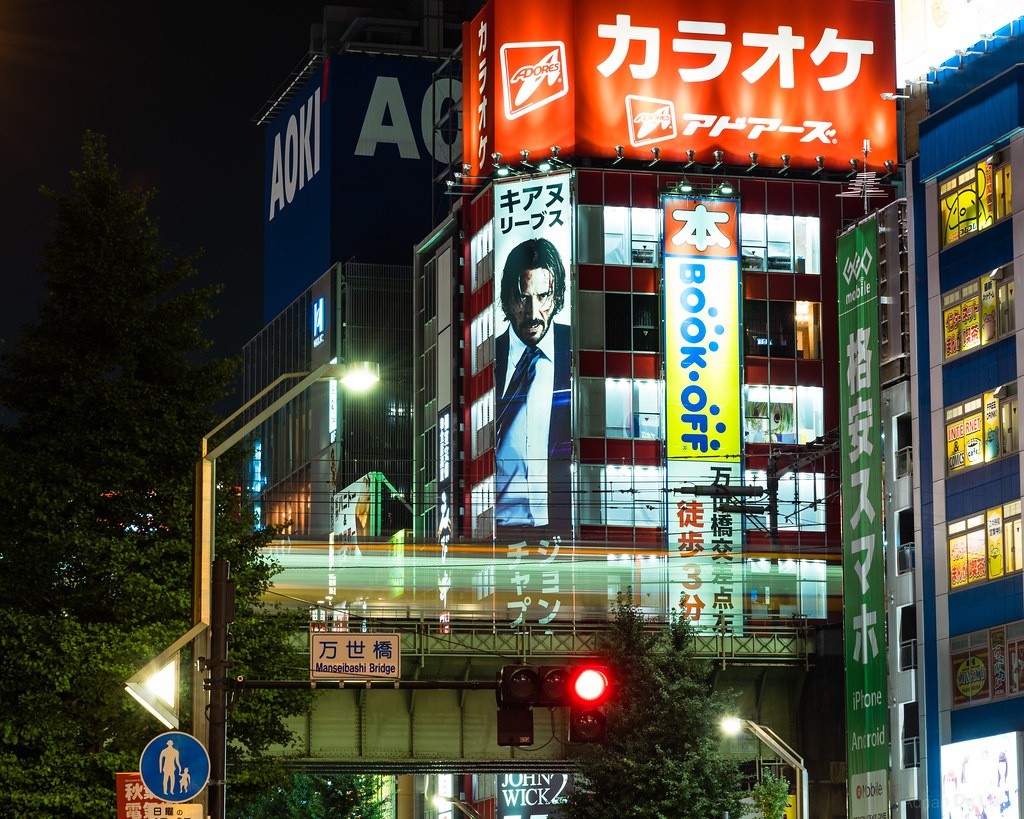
xmin=496 ymin=346 xmax=543 ymax=439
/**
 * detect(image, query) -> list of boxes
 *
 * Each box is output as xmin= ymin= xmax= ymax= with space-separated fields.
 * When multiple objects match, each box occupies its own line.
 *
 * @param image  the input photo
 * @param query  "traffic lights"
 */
xmin=498 ymin=664 xmax=612 ymax=742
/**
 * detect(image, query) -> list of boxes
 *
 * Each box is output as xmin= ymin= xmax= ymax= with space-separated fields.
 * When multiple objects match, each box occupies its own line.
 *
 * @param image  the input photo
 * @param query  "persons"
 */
xmin=496 ymin=238 xmax=572 ymax=528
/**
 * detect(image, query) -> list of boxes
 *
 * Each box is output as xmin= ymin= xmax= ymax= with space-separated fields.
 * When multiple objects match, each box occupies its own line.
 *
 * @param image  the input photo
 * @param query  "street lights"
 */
xmin=122 ymin=357 xmax=383 ymax=818
xmin=720 ymin=716 xmax=810 ymax=819
